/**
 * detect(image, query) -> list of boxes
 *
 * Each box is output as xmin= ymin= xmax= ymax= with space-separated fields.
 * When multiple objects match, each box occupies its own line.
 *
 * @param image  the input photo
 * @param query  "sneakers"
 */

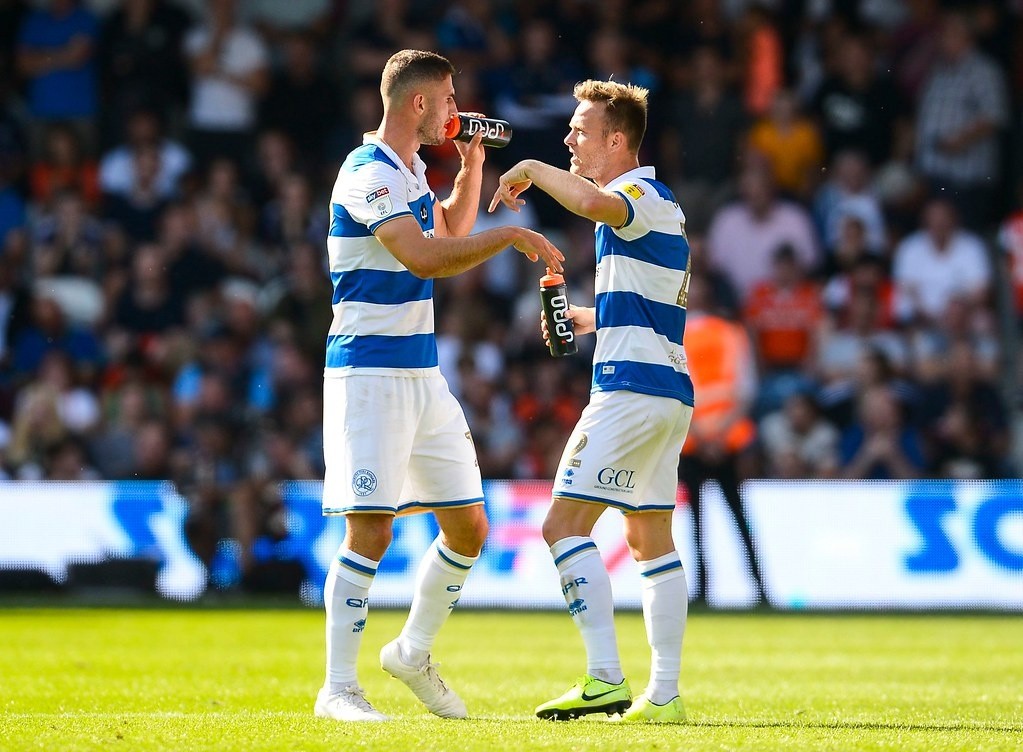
xmin=380 ymin=640 xmax=465 ymax=720
xmin=536 ymin=673 xmax=632 ymax=722
xmin=314 ymin=687 xmax=387 ymax=721
xmin=604 ymin=695 xmax=688 ymax=725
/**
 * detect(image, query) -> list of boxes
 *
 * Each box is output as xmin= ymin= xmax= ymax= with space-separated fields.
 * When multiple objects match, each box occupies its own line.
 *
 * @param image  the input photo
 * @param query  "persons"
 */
xmin=0 ymin=1 xmax=1023 ymax=606
xmin=490 ymin=80 xmax=695 ymax=723
xmin=314 ymin=49 xmax=566 ymax=719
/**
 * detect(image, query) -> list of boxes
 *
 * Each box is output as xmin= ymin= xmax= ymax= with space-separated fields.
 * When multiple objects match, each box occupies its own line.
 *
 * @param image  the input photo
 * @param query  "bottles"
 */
xmin=540 ymin=267 xmax=578 ymax=357
xmin=446 ymin=114 xmax=512 ymax=148
